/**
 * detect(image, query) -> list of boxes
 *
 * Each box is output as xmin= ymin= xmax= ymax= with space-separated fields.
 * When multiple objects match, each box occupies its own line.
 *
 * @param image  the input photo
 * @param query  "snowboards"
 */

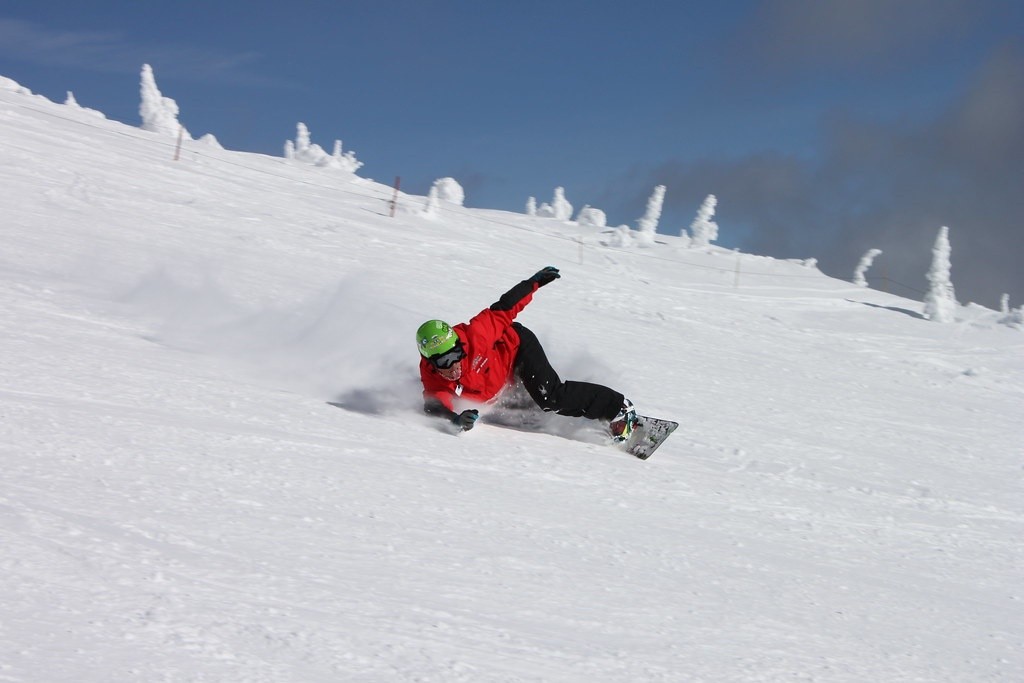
xmin=488 ymin=391 xmax=682 ymax=460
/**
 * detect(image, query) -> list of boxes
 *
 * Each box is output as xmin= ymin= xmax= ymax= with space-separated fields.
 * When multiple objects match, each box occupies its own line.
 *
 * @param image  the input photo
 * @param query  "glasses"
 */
xmin=435 ymin=351 xmax=462 ymax=368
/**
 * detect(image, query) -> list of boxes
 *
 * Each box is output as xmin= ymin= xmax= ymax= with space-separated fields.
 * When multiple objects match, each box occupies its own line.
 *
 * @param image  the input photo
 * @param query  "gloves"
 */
xmin=529 ymin=266 xmax=561 ymax=288
xmin=452 ymin=409 xmax=479 ymax=431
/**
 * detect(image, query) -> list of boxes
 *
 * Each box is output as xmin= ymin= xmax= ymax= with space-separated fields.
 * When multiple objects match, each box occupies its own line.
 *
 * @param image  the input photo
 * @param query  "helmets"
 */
xmin=416 ymin=320 xmax=468 ymax=370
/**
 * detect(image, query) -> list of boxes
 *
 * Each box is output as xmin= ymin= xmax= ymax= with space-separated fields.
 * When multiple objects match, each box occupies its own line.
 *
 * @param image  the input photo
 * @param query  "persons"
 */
xmin=415 ymin=266 xmax=637 ymax=441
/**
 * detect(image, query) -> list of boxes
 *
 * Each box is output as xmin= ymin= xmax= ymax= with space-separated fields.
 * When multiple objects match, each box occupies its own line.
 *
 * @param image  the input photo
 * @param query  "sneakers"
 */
xmin=610 ymin=398 xmax=638 ymax=443
